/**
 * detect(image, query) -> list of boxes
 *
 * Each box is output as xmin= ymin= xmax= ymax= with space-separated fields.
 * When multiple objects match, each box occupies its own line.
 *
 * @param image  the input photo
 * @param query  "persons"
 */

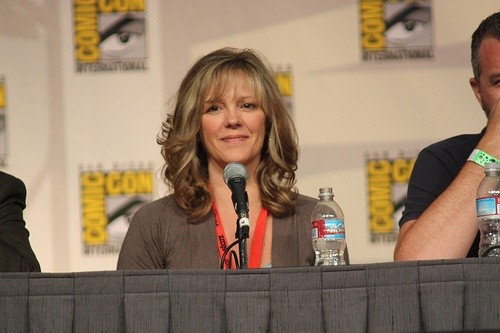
xmin=0 ymin=171 xmax=43 ymax=274
xmin=116 ymin=45 xmax=351 ymax=272
xmin=391 ymin=10 xmax=499 ymax=262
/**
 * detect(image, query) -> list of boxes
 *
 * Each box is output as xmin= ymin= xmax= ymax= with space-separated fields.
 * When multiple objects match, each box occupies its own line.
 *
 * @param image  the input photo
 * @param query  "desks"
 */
xmin=1 ymin=258 xmax=500 ymax=333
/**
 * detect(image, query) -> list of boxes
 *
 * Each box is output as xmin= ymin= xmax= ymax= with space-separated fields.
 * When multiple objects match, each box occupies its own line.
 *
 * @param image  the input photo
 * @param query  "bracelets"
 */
xmin=466 ymin=147 xmax=500 ymax=174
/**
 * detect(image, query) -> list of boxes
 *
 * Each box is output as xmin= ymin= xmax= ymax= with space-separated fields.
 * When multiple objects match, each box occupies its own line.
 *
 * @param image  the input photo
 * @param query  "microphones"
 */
xmin=223 ymin=161 xmax=250 ymax=238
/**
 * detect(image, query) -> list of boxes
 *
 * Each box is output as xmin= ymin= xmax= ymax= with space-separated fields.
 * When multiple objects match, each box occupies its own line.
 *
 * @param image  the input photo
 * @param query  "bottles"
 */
xmin=311 ymin=187 xmax=346 ymax=265
xmin=475 ymin=162 xmax=500 ymax=257
